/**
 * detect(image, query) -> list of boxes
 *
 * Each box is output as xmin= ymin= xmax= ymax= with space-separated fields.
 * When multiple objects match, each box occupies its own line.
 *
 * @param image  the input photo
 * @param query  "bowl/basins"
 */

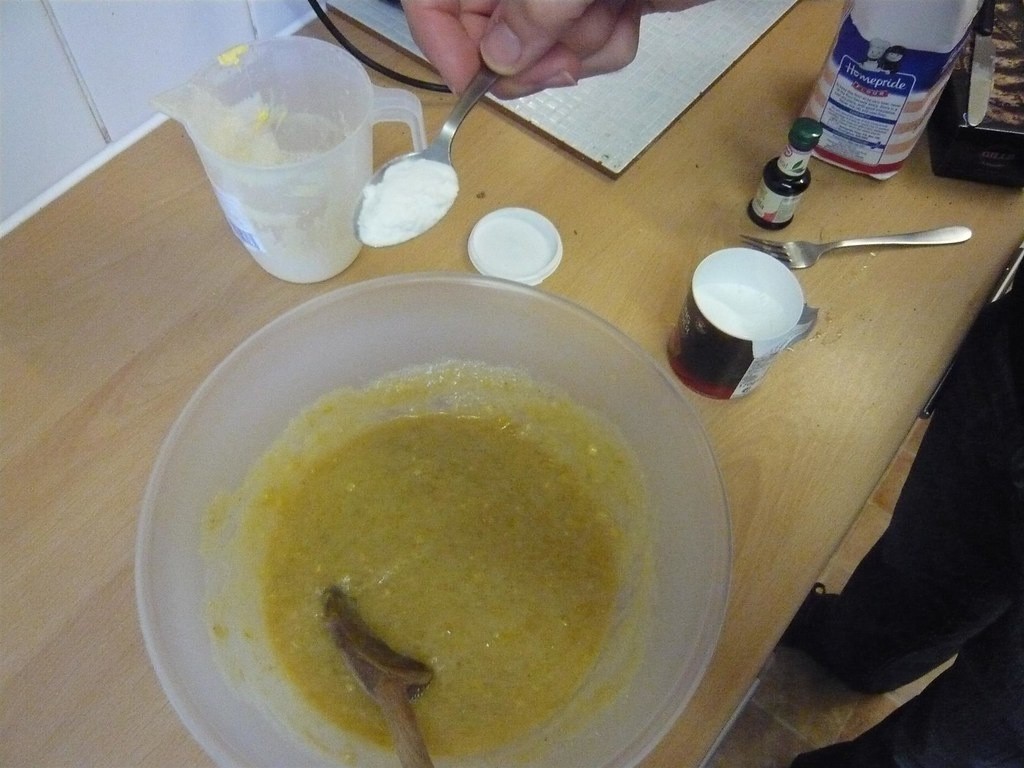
xmin=132 ymin=273 xmax=735 ymax=767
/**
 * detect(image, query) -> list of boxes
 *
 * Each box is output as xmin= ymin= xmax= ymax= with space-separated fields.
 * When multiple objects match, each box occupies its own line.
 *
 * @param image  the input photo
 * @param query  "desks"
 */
xmin=0 ymin=0 xmax=1024 ymax=768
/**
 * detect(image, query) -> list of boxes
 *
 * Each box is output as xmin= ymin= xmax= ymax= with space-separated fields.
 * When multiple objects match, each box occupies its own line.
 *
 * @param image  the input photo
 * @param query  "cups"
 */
xmin=666 ymin=246 xmax=804 ymax=399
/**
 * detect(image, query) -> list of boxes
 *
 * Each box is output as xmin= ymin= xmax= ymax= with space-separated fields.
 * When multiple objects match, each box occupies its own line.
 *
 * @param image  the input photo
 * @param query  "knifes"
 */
xmin=967 ymin=0 xmax=996 ymax=126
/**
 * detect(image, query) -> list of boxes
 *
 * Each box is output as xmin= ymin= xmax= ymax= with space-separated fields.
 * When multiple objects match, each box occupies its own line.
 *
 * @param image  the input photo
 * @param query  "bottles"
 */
xmin=801 ymin=1 xmax=983 ymax=181
xmin=748 ymin=117 xmax=824 ymax=230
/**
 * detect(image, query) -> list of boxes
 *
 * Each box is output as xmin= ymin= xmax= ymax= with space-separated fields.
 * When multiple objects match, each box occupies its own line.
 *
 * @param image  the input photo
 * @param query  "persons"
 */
xmin=402 ymin=0 xmax=1024 ymax=768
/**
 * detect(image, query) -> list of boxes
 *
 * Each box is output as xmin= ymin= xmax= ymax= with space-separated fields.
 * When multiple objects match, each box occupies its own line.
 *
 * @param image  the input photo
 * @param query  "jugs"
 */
xmin=150 ymin=36 xmax=427 ymax=284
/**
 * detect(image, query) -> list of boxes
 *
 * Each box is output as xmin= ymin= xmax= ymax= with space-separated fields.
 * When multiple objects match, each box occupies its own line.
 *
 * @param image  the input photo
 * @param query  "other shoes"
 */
xmin=776 ymin=581 xmax=895 ymax=696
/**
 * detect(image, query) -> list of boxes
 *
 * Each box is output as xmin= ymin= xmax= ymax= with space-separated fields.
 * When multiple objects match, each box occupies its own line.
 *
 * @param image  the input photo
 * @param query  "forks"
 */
xmin=739 ymin=225 xmax=973 ymax=267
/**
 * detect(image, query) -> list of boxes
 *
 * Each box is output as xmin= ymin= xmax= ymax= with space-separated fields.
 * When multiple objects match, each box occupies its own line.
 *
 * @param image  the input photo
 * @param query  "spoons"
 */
xmin=353 ymin=66 xmax=498 ymax=247
